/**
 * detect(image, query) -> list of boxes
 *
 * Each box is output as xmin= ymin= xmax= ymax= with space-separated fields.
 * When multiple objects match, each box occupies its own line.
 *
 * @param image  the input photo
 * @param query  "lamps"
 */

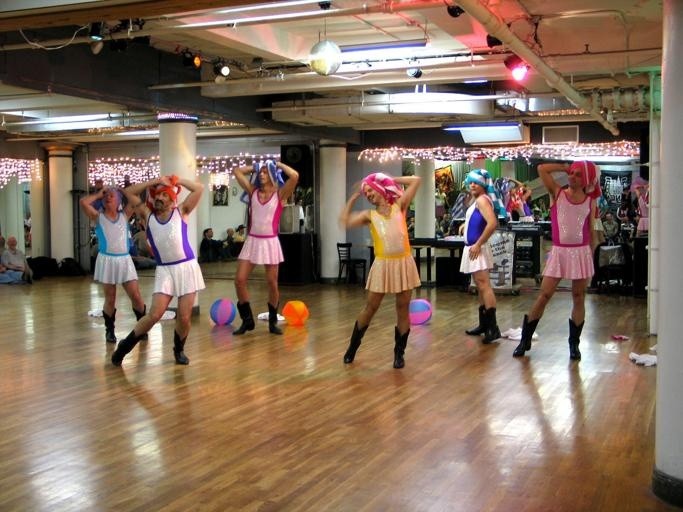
xmin=213 ymin=59 xmax=231 ymax=78
xmin=84 ymin=19 xmax=104 ymax=40
xmin=502 ymin=52 xmax=522 ymax=70
xmin=485 ymin=32 xmax=503 ymax=49
xmin=405 ymin=67 xmax=423 ymax=79
xmin=444 ymin=3 xmax=465 ymax=18
xmin=178 ymin=48 xmax=201 ymax=69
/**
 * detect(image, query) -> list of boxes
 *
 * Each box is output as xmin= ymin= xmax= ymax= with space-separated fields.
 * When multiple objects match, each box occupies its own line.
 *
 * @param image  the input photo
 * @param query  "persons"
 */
xmin=403 ymin=165 xmax=551 ymax=243
xmin=598 ymin=177 xmax=649 ymax=245
xmin=198 ymin=222 xmax=248 ymax=262
xmin=457 ymin=168 xmax=507 ymax=345
xmin=510 ymin=160 xmax=601 ymax=359
xmin=230 ymin=158 xmax=302 ymax=335
xmin=80 ymin=186 xmax=150 ymax=342
xmin=341 ymin=171 xmax=423 ymax=368
xmin=112 ymin=175 xmax=206 ymax=368
xmin=0 ymin=210 xmax=99 ymax=286
xmin=122 ymin=212 xmax=159 ymax=270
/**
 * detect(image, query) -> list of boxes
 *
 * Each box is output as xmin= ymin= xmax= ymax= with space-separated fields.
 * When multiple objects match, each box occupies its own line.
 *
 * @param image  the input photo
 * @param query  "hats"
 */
xmin=236 ymin=224 xmax=247 ymax=233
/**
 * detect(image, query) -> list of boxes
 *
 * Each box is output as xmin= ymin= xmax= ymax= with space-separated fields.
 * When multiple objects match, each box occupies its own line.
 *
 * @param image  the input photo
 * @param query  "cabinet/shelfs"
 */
xmin=497 ymin=225 xmax=543 ymax=284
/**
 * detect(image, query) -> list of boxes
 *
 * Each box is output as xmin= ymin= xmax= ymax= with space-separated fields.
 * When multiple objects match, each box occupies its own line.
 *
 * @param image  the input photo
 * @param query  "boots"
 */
xmin=131 ymin=304 xmax=148 ymax=340
xmin=465 ymin=305 xmax=487 ymax=336
xmin=513 ymin=314 xmax=540 ymax=358
xmin=267 ymin=301 xmax=283 ymax=335
xmin=567 ymin=318 xmax=585 ymax=361
xmin=232 ymin=300 xmax=256 ymax=336
xmin=343 ymin=319 xmax=369 ymax=364
xmin=102 ymin=307 xmax=116 ymax=343
xmin=481 ymin=306 xmax=502 ymax=344
xmin=392 ymin=325 xmax=410 ymax=369
xmin=111 ymin=329 xmax=142 ymax=367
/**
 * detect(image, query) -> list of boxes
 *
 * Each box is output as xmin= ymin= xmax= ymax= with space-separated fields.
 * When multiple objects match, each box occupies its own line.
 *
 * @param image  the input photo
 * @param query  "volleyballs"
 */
xmin=409 ymin=299 xmax=432 ymax=325
xmin=210 ymin=298 xmax=236 ymax=326
xmin=282 ymin=301 xmax=308 ymax=325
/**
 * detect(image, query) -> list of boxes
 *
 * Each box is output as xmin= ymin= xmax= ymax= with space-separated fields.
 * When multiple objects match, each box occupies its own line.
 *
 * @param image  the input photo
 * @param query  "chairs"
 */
xmin=336 ymin=241 xmax=368 ymax=291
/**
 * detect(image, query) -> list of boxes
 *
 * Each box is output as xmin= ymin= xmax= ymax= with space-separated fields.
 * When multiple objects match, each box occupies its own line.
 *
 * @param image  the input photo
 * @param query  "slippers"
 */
xmin=172 ymin=329 xmax=190 ymax=364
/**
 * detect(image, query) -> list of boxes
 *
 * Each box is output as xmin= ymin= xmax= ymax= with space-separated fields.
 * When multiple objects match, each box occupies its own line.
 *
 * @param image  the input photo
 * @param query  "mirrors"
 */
xmin=399 ymin=148 xmax=642 ymax=243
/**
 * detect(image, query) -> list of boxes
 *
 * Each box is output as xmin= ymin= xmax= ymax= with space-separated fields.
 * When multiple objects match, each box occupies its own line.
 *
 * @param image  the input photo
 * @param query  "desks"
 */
xmin=365 ymin=243 xmax=433 ymax=287
